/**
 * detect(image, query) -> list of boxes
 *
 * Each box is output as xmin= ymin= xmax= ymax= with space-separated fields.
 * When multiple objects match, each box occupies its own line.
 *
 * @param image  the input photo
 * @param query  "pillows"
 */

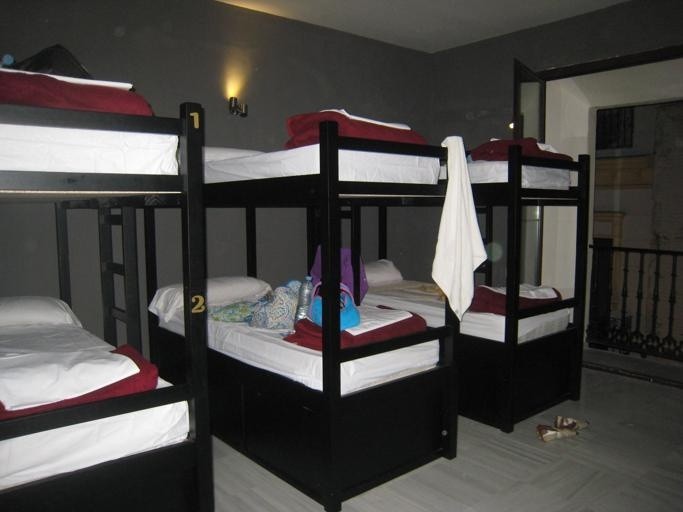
xmin=363 ymin=258 xmax=405 ymax=291
xmin=1 ymin=294 xmax=78 ymax=336
xmin=148 ymin=275 xmax=272 ymax=324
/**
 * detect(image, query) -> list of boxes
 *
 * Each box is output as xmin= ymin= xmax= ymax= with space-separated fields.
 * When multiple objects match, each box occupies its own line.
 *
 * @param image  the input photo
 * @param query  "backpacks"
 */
xmin=310 ymin=281 xmax=360 ymax=331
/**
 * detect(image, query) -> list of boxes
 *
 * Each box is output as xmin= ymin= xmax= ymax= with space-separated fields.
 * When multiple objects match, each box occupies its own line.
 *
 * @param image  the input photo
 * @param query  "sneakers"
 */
xmin=536 ymin=416 xmax=589 ymax=442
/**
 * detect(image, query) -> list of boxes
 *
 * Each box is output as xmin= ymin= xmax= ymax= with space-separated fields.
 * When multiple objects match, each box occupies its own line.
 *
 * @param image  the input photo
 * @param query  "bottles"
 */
xmin=295 ymin=275 xmax=313 ymax=327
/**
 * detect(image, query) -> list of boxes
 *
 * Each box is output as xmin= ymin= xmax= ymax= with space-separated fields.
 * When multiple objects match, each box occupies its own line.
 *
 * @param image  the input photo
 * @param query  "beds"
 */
xmin=143 ymin=116 xmax=459 ymax=508
xmin=307 ymin=112 xmax=588 ymax=434
xmin=0 ymin=99 xmax=216 ymax=512
